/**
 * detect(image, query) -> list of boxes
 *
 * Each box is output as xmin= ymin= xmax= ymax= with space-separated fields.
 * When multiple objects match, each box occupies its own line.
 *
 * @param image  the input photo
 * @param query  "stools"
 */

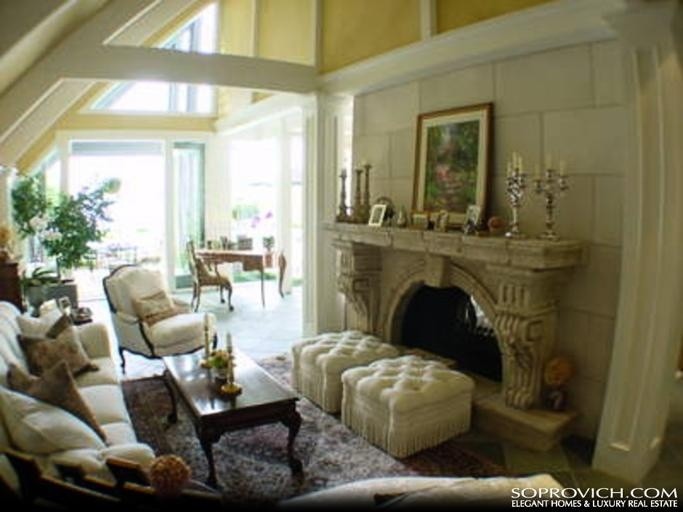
xmin=340 ymin=355 xmax=474 ymax=459
xmin=290 ymin=330 xmax=400 ymax=413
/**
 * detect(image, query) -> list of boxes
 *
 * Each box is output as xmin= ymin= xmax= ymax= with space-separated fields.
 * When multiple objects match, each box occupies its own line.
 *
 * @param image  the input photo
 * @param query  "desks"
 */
xmin=193 ymin=249 xmax=287 ymax=309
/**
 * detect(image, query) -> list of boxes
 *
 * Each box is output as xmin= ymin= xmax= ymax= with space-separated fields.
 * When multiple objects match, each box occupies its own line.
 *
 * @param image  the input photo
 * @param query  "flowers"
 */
xmin=8 ymin=171 xmax=121 ymax=271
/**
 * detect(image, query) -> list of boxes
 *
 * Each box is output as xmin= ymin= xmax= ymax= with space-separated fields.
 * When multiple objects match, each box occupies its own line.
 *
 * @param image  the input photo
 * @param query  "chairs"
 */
xmin=185 ymin=240 xmax=234 ymax=314
xmin=102 ymin=264 xmax=219 ymax=374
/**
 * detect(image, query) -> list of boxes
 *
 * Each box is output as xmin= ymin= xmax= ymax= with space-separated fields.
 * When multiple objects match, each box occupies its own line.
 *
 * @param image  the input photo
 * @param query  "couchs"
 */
xmin=0 ymin=301 xmax=157 ymax=499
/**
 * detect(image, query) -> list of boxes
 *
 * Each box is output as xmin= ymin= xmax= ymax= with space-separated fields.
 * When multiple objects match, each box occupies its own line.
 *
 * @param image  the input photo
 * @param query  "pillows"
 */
xmin=130 ymin=286 xmax=181 ymax=328
xmin=0 ymin=293 xmax=111 ymax=455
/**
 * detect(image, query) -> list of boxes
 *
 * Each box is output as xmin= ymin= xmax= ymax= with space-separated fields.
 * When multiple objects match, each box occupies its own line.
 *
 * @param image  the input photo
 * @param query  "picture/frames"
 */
xmin=410 ymin=101 xmax=493 ymax=230
xmin=368 ymin=204 xmax=386 ymax=227
xmin=463 ymin=204 xmax=481 ymax=233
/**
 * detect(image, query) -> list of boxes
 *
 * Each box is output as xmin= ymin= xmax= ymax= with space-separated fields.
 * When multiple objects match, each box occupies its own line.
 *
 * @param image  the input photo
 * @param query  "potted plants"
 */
xmin=18 ymin=265 xmax=58 ymax=318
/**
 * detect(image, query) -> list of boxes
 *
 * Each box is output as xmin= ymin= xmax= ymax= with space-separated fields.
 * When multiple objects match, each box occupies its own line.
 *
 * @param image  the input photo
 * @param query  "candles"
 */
xmin=339 ymin=168 xmax=346 ymax=176
xmin=201 ymin=311 xmax=211 ymax=332
xmin=506 ymin=151 xmax=567 ymax=180
xmin=225 ymin=331 xmax=234 ymax=352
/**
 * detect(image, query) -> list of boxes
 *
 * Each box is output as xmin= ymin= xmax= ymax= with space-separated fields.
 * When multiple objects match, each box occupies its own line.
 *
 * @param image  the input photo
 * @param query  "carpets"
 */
xmin=120 ymin=356 xmax=519 ymax=504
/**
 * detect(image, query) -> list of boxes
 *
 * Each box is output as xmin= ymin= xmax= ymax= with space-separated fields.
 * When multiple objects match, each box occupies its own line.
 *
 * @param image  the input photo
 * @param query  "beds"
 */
xmin=163 ymin=356 xmax=303 ymax=485
